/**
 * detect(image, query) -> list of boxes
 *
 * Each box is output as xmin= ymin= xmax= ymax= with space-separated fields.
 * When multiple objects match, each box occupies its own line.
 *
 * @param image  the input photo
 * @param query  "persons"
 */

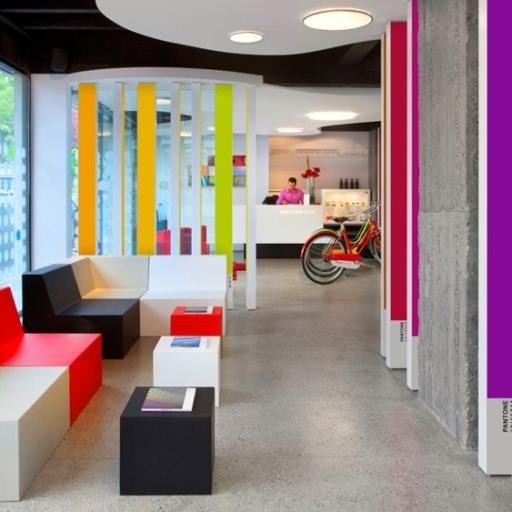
xmin=276 ymin=177 xmax=304 ymax=205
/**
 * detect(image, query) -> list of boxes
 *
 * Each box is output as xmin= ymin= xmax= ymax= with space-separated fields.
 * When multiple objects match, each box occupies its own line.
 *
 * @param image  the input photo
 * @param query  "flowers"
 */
xmin=301 ymin=167 xmax=320 ymax=179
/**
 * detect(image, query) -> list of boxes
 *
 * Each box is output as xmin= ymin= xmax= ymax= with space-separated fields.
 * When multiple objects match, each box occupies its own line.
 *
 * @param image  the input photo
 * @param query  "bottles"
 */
xmin=338 ymin=176 xmax=360 ymax=189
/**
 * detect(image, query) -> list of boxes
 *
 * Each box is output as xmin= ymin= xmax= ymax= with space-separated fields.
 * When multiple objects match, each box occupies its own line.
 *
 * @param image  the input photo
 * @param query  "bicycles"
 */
xmin=300 ymin=202 xmax=381 ymax=284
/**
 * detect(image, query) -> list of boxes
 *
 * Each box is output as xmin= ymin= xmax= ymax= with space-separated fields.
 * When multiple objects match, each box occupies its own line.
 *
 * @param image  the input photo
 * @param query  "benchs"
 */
xmin=0 ymin=285 xmax=103 ymax=503
xmin=21 ymin=254 xmax=229 ymax=360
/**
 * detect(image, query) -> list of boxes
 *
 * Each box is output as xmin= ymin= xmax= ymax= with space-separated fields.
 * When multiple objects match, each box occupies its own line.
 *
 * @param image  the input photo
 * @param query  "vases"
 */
xmin=307 ymin=177 xmax=315 ymax=204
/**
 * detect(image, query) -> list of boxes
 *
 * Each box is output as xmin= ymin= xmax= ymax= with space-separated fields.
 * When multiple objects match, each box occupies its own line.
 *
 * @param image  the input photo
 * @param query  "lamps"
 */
xmin=180 ymin=131 xmax=192 ymax=137
xmin=228 ymin=30 xmax=264 ymax=44
xmin=156 ymin=98 xmax=171 ymax=106
xmin=302 ymin=8 xmax=374 ymax=32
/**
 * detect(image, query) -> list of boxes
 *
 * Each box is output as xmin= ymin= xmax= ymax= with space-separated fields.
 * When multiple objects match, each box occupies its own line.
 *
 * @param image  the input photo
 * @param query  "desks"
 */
xmin=119 ymin=386 xmax=215 ymax=495
xmin=153 ymin=335 xmax=222 ymax=407
xmin=170 ymin=305 xmax=223 ymax=355
